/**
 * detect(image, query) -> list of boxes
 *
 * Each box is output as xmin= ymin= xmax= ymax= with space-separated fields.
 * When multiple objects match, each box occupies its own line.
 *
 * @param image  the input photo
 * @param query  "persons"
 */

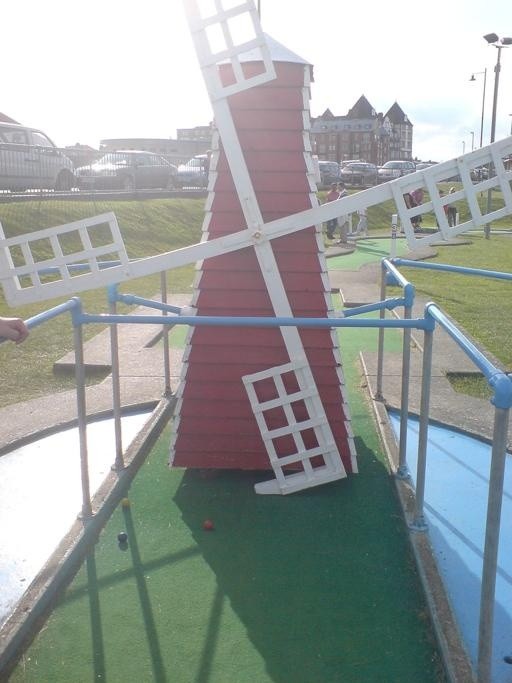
xmin=403 ymin=186 xmax=457 ymax=233
xmin=0 ymin=316 xmax=29 ymax=344
xmin=326 ymin=181 xmax=367 ymax=242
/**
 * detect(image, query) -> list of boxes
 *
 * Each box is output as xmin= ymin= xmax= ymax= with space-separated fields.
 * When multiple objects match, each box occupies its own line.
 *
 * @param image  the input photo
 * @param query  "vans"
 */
xmin=0 ymin=122 xmax=76 ymax=193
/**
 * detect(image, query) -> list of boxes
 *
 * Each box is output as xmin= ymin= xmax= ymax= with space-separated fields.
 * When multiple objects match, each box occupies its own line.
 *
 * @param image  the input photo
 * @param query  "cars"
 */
xmin=176 ymin=151 xmax=211 ymax=188
xmin=77 ymin=149 xmax=179 ymax=192
xmin=319 ymin=161 xmax=437 ymax=185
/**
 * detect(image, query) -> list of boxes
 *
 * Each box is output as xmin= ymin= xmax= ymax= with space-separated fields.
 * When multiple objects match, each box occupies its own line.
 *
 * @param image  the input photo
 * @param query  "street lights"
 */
xmin=469 ymin=67 xmax=488 ymax=148
xmin=470 ymin=131 xmax=475 ymax=152
xmin=462 ymin=141 xmax=466 ymax=155
xmin=484 ymin=31 xmax=512 ymax=145
xmin=508 ymin=113 xmax=512 ymax=136
